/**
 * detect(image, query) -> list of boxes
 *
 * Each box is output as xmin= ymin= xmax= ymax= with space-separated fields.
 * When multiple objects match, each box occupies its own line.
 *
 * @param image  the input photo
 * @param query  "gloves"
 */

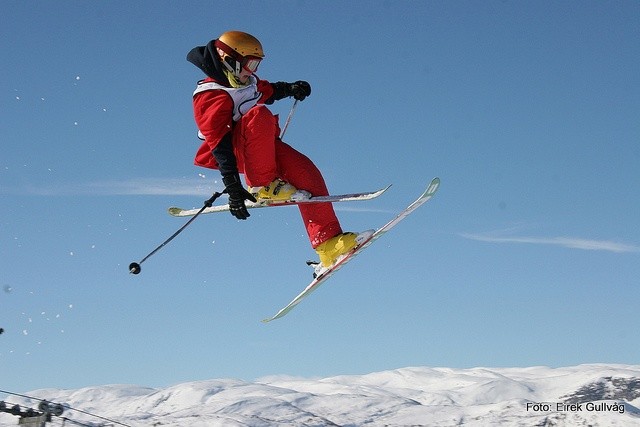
xmin=221 ymin=171 xmax=256 ymax=220
xmin=288 ymin=80 xmax=311 ymax=103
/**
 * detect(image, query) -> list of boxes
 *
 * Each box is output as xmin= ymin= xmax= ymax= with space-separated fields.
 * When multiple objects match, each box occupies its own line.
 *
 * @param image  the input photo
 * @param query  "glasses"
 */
xmin=243 ymin=55 xmax=263 ymax=73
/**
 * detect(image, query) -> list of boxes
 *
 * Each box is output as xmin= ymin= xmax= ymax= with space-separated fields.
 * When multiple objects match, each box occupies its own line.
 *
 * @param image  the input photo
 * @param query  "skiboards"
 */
xmin=169 ymin=178 xmax=439 ymax=323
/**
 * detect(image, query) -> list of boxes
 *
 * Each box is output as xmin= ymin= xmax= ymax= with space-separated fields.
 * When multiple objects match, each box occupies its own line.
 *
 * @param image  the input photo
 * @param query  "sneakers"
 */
xmin=316 ymin=232 xmax=357 ymax=268
xmin=247 ymin=176 xmax=297 ymax=201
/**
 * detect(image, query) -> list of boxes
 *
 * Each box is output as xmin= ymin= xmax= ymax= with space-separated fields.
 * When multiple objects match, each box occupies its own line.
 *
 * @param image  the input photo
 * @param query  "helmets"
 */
xmin=216 ymin=32 xmax=265 ymax=88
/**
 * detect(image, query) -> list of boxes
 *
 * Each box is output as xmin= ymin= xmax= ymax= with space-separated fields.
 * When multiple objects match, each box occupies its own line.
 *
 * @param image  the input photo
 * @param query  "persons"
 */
xmin=180 ymin=28 xmax=375 ymax=276
